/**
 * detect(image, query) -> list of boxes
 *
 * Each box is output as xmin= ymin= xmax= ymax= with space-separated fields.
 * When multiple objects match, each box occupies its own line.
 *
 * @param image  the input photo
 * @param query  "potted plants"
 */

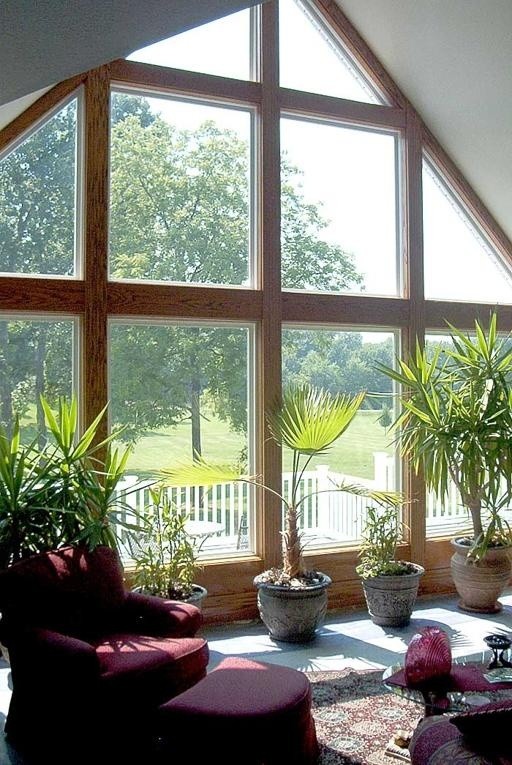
xmin=132 ymin=379 xmax=424 ymax=644
xmin=352 ymin=497 xmax=427 ymax=627
xmin=123 ymin=484 xmax=212 ymax=644
xmin=364 ymin=301 xmax=512 ymax=617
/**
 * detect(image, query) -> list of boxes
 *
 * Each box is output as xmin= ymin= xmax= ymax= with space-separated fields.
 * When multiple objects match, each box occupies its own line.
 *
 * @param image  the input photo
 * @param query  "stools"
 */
xmin=161 ymin=655 xmax=319 ymax=765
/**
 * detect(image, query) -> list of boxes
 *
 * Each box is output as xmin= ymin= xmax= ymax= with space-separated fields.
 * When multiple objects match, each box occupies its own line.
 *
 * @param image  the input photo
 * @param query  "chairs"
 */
xmin=0 ymin=543 xmax=211 ymax=765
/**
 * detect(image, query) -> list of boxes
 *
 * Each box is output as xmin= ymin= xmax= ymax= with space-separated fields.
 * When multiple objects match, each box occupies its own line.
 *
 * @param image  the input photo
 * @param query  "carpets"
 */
xmin=295 ymin=663 xmax=510 ymax=764
